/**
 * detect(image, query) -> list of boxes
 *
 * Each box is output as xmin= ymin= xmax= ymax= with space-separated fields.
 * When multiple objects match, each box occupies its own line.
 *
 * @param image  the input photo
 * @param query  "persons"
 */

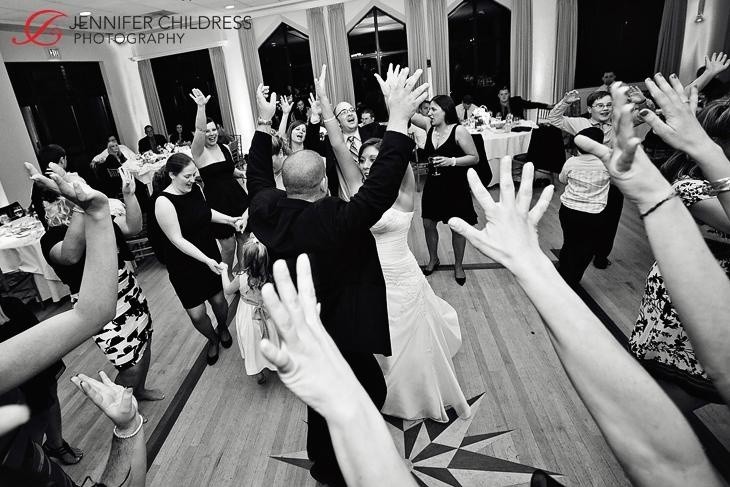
xmin=0 ymin=52 xmax=730 ymax=487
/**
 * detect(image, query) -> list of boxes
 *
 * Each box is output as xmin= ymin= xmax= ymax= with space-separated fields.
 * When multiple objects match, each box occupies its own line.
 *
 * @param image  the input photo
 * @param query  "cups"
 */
xmin=461 ymin=111 xmax=520 ymax=134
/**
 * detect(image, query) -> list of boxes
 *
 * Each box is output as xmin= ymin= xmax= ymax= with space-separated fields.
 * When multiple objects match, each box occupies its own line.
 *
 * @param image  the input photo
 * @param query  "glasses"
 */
xmin=591 ymin=103 xmax=614 ymax=109
xmin=336 ymin=108 xmax=356 ymax=118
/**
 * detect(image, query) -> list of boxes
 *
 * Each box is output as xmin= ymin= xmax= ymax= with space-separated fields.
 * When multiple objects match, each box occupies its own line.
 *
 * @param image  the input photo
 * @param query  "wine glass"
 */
xmin=429 ymin=157 xmax=442 ymax=177
xmin=1 ymin=207 xmax=44 ymax=239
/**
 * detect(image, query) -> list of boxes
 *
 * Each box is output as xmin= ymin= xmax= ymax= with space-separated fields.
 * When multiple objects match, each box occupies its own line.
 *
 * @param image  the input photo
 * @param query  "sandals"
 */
xmin=43 ymin=438 xmax=85 ymax=464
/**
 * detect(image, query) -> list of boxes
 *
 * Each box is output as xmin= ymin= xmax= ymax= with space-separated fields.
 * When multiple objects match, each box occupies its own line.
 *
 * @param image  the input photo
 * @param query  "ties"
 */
xmin=465 ymin=109 xmax=467 ymax=120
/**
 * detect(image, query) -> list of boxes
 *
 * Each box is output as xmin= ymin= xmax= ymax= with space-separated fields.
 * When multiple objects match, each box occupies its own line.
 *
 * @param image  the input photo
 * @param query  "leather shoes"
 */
xmin=423 ymin=259 xmax=441 ymax=276
xmin=221 ymin=333 xmax=232 ymax=348
xmin=206 ymin=344 xmax=219 ymax=366
xmin=455 ymin=266 xmax=466 ymax=286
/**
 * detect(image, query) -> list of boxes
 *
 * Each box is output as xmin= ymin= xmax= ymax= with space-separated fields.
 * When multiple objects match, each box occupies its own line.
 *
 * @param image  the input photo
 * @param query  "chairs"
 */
xmin=2 ymin=85 xmax=574 ymax=325
